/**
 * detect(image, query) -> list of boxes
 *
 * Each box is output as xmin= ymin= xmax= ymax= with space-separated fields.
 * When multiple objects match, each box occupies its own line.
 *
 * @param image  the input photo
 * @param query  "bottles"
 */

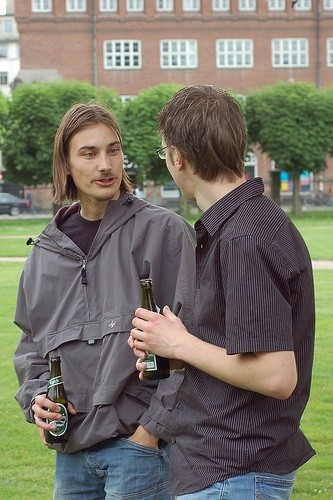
xmin=137 ymin=278 xmax=170 ymax=379
xmin=45 ymin=355 xmax=70 ymax=443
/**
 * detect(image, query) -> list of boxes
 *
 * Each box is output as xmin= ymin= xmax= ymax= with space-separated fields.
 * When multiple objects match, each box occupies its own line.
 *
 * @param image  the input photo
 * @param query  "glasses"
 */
xmin=156 ymin=146 xmax=169 ymax=159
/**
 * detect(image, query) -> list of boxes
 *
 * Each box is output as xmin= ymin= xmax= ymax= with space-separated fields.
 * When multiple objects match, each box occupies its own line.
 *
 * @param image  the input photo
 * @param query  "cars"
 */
xmin=0 ymin=193 xmax=30 ymax=216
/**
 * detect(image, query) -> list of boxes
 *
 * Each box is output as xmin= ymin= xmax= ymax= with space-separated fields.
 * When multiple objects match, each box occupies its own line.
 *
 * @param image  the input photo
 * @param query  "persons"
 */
xmin=18 ymin=187 xmax=37 ymax=215
xmin=127 ymin=83 xmax=317 ymax=500
xmin=14 ymin=99 xmax=196 ymax=500
